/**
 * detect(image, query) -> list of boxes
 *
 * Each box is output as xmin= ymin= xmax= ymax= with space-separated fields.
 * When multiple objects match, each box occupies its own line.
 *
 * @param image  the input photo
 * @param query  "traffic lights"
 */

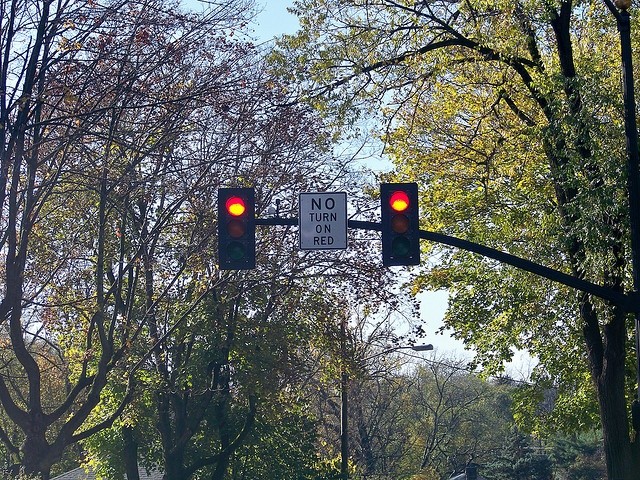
xmin=218 ymin=187 xmax=256 ymax=270
xmin=381 ymin=183 xmax=420 ymax=266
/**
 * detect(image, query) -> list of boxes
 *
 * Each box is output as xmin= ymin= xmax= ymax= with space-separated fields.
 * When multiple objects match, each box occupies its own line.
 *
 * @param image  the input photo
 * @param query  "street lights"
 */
xmin=339 ymin=344 xmax=434 ymax=480
